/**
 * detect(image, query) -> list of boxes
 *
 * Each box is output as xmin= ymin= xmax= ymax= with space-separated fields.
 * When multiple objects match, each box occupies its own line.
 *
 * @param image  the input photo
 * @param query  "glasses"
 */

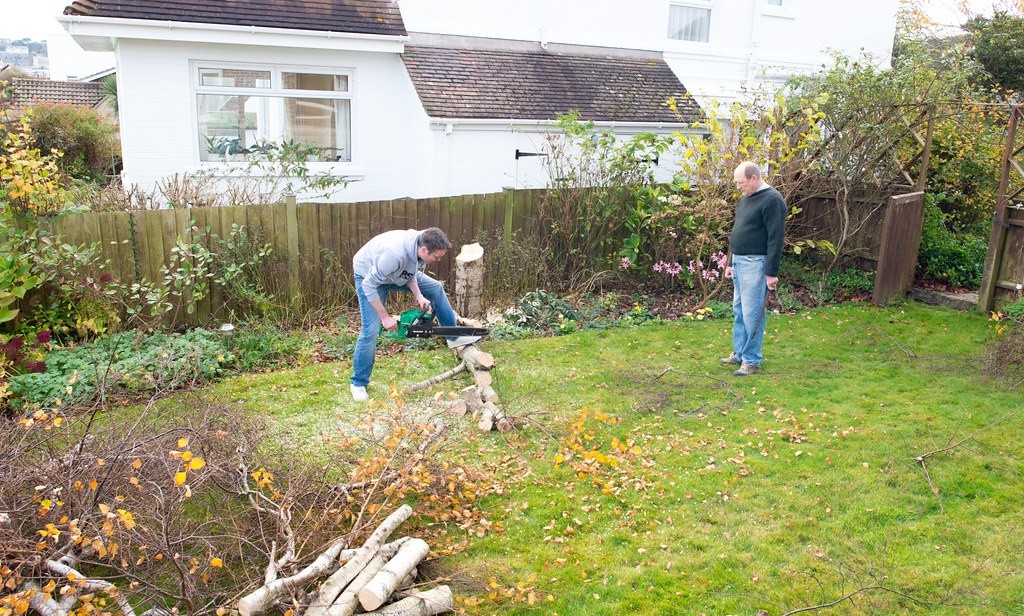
xmin=431 ymin=253 xmax=441 ymax=262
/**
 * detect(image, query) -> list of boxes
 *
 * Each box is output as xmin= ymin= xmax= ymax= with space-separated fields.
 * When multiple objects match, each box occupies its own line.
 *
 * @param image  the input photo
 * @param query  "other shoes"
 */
xmin=733 ymin=362 xmax=759 ymax=376
xmin=720 ymin=351 xmax=740 ymax=364
xmin=446 ymin=336 xmax=482 ymax=349
xmin=350 ymin=384 xmax=369 ymax=402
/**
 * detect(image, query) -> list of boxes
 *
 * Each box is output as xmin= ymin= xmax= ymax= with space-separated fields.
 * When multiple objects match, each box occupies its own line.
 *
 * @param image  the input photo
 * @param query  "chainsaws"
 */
xmin=378 ymin=303 xmax=490 ymax=341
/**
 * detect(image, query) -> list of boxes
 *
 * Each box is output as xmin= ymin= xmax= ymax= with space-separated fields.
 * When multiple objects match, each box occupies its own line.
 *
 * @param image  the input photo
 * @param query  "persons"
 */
xmin=719 ymin=161 xmax=788 ymax=375
xmin=349 ymin=227 xmax=482 ymax=402
